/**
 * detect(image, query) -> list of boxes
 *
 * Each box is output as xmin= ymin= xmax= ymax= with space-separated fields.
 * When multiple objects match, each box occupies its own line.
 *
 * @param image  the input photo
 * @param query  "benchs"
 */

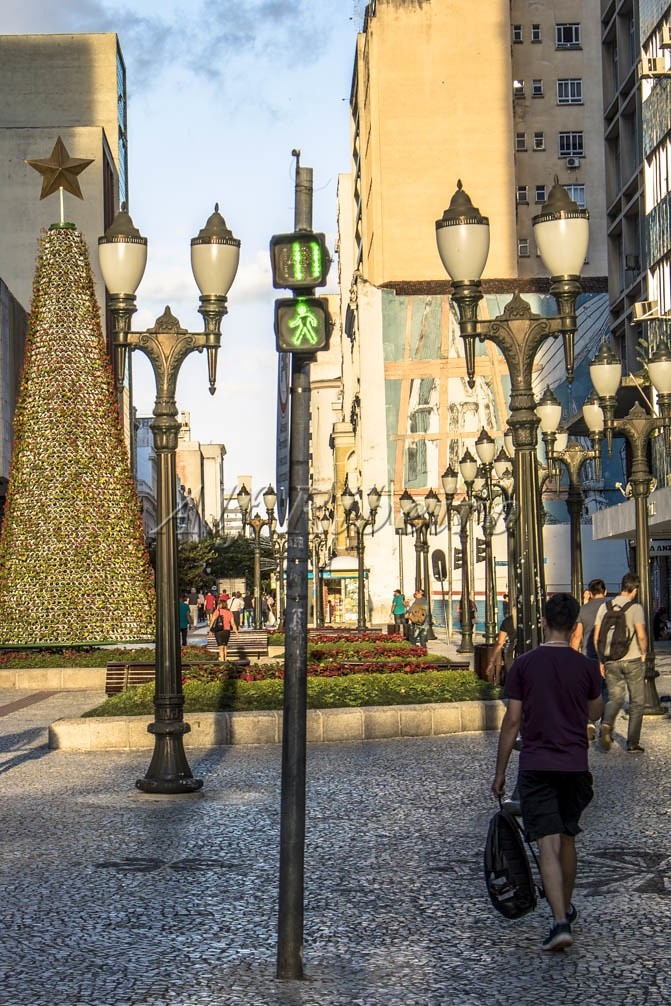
xmin=308 ymin=628 xmax=382 ymax=637
xmin=339 ymin=662 xmax=471 ymax=671
xmin=105 ymin=661 xmax=251 ymax=698
xmin=206 ymin=630 xmax=268 ymax=658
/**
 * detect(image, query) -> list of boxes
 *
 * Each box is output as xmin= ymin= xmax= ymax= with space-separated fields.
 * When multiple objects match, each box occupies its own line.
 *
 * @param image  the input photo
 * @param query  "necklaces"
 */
xmin=546 ymin=640 xmax=568 ymax=645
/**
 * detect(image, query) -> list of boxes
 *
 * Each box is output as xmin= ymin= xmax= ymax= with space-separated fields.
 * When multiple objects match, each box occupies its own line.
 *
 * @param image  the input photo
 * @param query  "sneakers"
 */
xmin=541 ymin=923 xmax=573 ymax=951
xmin=565 ymin=902 xmax=579 ymax=925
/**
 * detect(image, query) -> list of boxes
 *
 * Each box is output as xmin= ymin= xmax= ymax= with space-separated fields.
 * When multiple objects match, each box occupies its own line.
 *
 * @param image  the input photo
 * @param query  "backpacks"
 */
xmin=409 ymin=602 xmax=427 ymax=626
xmin=484 ymin=796 xmax=545 ymax=920
xmin=597 ymin=599 xmax=636 ymax=665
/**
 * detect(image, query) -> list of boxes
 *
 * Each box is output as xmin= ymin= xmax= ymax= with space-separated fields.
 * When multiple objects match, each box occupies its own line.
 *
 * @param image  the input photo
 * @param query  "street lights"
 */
xmin=434 ymin=172 xmax=594 ymax=655
xmin=340 ymin=483 xmax=382 ymax=634
xmin=589 ymin=338 xmax=671 ymax=717
xmin=95 ymin=198 xmax=242 ymax=795
xmin=267 ymin=515 xmax=287 ymax=631
xmin=398 ymin=420 xmax=569 ymax=657
xmin=307 ymin=506 xmax=331 ymax=627
xmin=534 ymin=383 xmax=606 ymax=606
xmin=236 ymin=482 xmax=278 ymax=632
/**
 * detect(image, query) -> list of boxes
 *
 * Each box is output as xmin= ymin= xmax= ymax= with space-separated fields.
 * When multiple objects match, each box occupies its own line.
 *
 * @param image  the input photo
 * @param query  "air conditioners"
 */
xmin=566 ymin=158 xmax=580 ymax=167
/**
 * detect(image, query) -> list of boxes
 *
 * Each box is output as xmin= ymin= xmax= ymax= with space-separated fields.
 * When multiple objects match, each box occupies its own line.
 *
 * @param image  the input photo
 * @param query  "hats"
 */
xmin=393 ymin=589 xmax=401 ymax=594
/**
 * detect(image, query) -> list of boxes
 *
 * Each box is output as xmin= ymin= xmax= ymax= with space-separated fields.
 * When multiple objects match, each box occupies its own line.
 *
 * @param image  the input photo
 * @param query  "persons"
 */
xmin=391 ymin=589 xmax=429 ymax=649
xmin=491 ymin=592 xmax=604 ymax=950
xmin=456 ymin=573 xmax=648 ymax=753
xmin=179 ymin=587 xmax=273 ymax=662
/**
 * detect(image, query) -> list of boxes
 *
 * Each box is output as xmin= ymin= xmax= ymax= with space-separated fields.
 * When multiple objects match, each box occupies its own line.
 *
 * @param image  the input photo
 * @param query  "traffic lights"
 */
xmin=272 ymin=296 xmax=334 ymax=352
xmin=269 ymin=230 xmax=330 ymax=289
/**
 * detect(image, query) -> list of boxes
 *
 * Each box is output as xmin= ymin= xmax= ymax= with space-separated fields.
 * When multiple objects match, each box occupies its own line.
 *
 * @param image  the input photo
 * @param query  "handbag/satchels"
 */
xmin=471 ymin=608 xmax=480 ymax=624
xmin=210 ymin=609 xmax=224 ymax=633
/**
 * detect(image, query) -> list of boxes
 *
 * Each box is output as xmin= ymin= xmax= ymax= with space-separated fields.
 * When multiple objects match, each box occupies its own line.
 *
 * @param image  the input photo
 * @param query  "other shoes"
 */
xmin=586 ymin=722 xmax=596 ymax=741
xmin=626 ymin=745 xmax=644 ymax=753
xmin=600 ymin=724 xmax=612 ymax=752
